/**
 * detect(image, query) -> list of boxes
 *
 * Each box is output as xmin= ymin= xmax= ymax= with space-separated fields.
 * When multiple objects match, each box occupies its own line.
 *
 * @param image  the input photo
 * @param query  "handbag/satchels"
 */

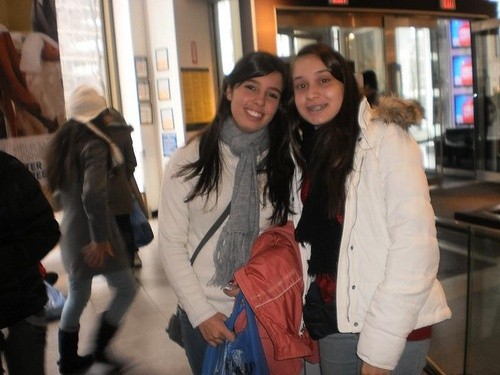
xmin=167 ymin=316 xmax=184 ymax=348
xmin=201 ymin=295 xmax=272 ymax=375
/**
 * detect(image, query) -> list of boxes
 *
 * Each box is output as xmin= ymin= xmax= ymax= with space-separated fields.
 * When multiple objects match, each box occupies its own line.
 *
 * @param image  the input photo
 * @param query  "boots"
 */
xmin=56 ymin=324 xmax=94 ymax=375
xmin=92 ymin=312 xmax=121 ymax=366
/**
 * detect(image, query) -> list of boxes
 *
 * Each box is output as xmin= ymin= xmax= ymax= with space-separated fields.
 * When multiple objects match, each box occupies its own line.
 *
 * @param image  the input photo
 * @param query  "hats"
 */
xmin=68 ymin=85 xmax=124 ymax=167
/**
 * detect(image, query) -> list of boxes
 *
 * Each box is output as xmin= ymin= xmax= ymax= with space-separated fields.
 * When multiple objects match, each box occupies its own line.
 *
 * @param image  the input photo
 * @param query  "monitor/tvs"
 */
xmin=450 ymin=18 xmax=472 ymax=49
xmin=452 ymin=54 xmax=473 ymax=87
xmin=454 ymin=93 xmax=474 ymax=125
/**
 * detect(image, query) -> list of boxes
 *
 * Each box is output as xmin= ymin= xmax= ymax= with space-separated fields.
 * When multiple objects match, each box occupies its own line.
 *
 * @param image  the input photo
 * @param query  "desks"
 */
xmin=454 ymin=201 xmax=500 ymax=375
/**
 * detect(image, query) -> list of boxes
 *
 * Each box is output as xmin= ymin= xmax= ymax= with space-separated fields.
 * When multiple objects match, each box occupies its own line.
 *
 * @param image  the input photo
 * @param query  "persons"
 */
xmin=44 ymin=90 xmax=136 ymax=375
xmin=0 ymin=151 xmax=62 ymax=375
xmin=103 ymin=107 xmax=141 ymax=267
xmin=157 ymin=51 xmax=288 ymax=375
xmin=287 ymin=43 xmax=452 ymax=375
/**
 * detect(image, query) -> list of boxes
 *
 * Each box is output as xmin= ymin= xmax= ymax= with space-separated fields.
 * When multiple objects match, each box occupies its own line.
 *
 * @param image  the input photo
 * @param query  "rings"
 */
xmin=220 ymin=336 xmax=225 ymax=340
